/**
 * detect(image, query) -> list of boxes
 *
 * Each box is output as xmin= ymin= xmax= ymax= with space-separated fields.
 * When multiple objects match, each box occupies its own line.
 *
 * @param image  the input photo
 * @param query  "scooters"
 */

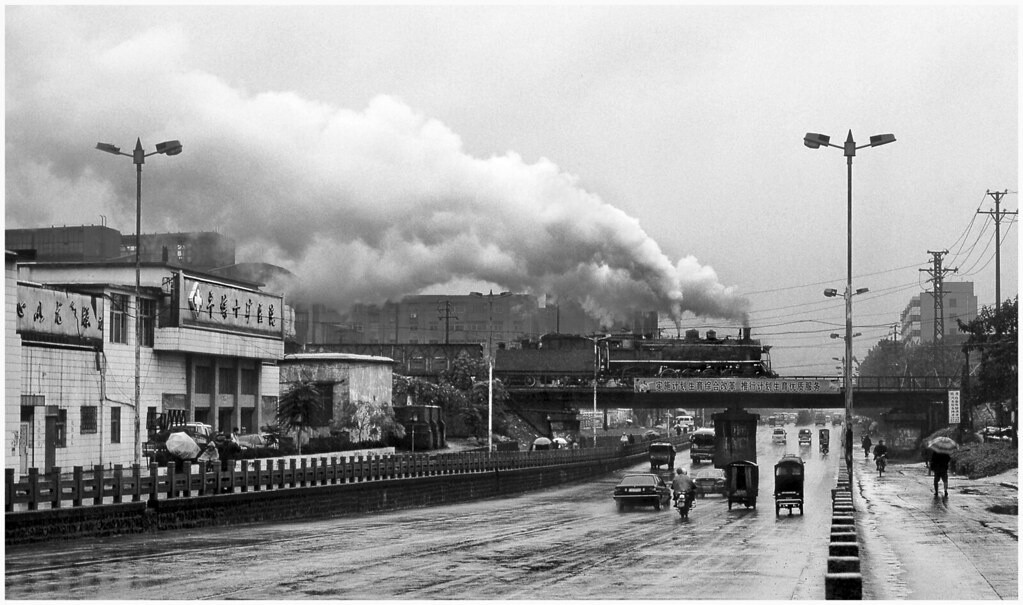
xmin=823 ymin=446 xmax=829 ymax=455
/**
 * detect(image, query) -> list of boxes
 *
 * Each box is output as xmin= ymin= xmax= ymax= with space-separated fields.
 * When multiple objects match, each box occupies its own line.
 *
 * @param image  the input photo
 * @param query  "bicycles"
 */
xmin=875 ymin=454 xmax=886 ymax=477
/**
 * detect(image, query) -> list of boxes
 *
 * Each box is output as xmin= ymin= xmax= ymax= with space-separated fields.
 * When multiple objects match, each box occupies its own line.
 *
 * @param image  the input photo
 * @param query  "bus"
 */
xmin=689 ymin=427 xmax=715 ymax=464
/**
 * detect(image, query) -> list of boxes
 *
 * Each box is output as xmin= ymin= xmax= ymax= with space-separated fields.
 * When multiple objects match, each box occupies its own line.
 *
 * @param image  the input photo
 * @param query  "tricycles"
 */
xmin=726 ymin=459 xmax=760 ymax=511
xmin=774 ymin=456 xmax=806 ymax=516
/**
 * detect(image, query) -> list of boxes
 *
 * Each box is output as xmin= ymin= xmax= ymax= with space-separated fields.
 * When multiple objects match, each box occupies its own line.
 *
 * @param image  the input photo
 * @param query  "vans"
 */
xmin=673 ymin=415 xmax=693 ymax=425
xmin=649 ymin=442 xmax=675 ymax=470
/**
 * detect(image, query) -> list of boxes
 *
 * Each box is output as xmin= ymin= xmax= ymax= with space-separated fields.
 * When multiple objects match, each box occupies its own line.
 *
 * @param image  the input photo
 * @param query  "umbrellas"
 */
xmin=533 ymin=437 xmax=552 ymax=445
xmin=553 ymin=438 xmax=567 ymax=444
xmin=928 ymin=436 xmax=959 ymax=454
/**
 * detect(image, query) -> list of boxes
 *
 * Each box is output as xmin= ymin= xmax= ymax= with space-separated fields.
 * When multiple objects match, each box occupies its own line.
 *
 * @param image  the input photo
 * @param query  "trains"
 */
xmin=306 ymin=326 xmax=773 ymax=387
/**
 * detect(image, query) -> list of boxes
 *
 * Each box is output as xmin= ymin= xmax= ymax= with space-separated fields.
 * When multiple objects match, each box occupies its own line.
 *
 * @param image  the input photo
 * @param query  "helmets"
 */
xmin=682 ymin=468 xmax=687 ymax=475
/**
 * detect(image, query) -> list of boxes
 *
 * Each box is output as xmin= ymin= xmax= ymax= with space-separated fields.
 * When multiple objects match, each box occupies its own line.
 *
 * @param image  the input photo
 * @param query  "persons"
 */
xmin=873 ymin=440 xmax=888 ymax=473
xmin=552 ymin=440 xmax=559 ymax=450
xmin=620 ymin=432 xmax=628 ymax=445
xmin=629 ymin=434 xmax=635 ymax=444
xmin=674 ymin=423 xmax=696 ymax=435
xmin=672 ymin=467 xmax=696 ymax=508
xmin=930 ymin=451 xmax=948 ymax=496
xmin=821 ymin=437 xmax=829 ymax=453
xmin=164 ymin=427 xmax=243 ymax=499
xmin=861 ymin=434 xmax=872 ymax=457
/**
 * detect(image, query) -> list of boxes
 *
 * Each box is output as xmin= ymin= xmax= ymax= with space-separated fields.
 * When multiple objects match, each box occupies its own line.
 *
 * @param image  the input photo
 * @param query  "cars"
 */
xmin=798 ymin=429 xmax=813 ymax=446
xmin=612 ymin=473 xmax=672 ymax=513
xmin=142 ymin=424 xmax=214 ymax=458
xmin=771 ymin=428 xmax=788 ymax=446
xmin=692 ymin=468 xmax=726 ymax=498
xmin=804 ymin=129 xmax=897 ymax=485
xmin=769 ymin=412 xmax=842 ymax=426
xmin=818 ymin=429 xmax=829 ymax=444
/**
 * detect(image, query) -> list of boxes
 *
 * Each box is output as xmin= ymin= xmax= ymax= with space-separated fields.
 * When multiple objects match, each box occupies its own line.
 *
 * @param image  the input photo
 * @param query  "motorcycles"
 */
xmin=671 ymin=486 xmax=697 ymax=519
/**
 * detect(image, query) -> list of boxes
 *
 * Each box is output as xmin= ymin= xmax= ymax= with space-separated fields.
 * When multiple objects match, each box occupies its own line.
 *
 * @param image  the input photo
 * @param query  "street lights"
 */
xmin=579 ymin=333 xmax=611 ymax=450
xmin=95 ymin=137 xmax=186 ymax=466
xmin=468 ymin=289 xmax=514 ymax=459
